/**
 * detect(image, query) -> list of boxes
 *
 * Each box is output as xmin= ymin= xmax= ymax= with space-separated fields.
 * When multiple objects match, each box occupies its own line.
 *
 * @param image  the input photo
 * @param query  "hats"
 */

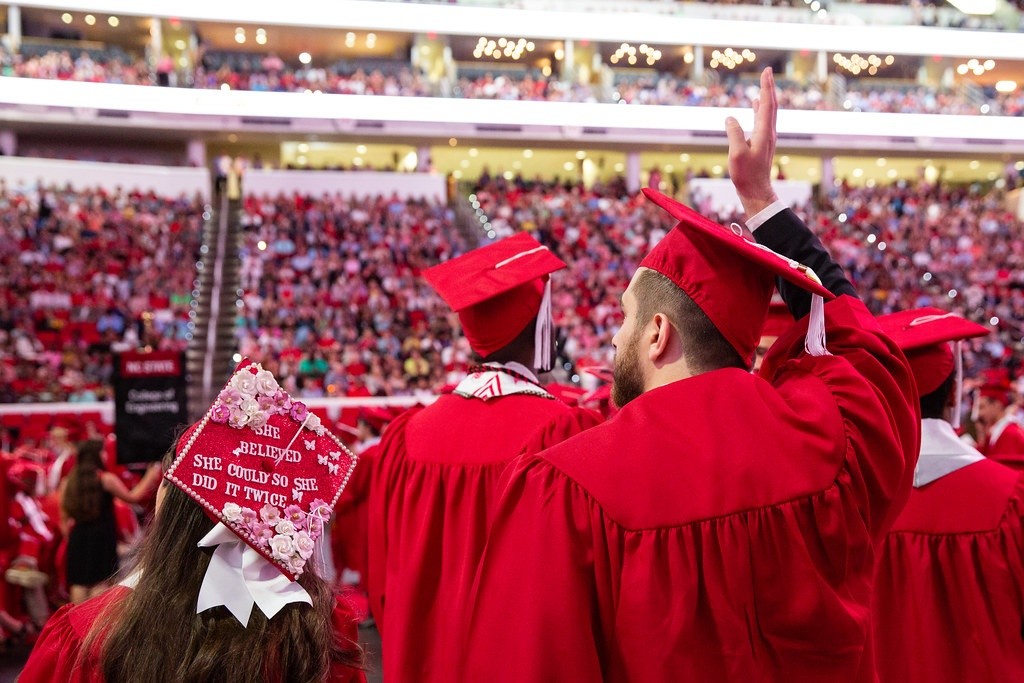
xmin=162 ymin=356 xmax=361 ymax=626
xmin=637 ymin=187 xmax=835 ymax=369
xmin=872 ymin=305 xmax=992 ymax=436
xmin=421 ymin=230 xmax=567 ymax=370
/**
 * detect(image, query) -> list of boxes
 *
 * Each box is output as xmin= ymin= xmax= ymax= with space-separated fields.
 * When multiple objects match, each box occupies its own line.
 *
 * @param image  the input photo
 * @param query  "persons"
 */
xmin=856 ymin=306 xmax=1023 ymax=682
xmin=216 ymin=149 xmax=473 ymax=682
xmin=2 ymin=47 xmax=1024 ymax=116
xmin=473 ymin=63 xmax=923 ymax=683
xmin=476 ymin=164 xmax=1024 ymax=472
xmin=2 ymin=178 xmax=204 ymax=638
xmin=19 ymin=355 xmax=371 ymax=682
xmin=377 ymin=234 xmax=579 ymax=683
